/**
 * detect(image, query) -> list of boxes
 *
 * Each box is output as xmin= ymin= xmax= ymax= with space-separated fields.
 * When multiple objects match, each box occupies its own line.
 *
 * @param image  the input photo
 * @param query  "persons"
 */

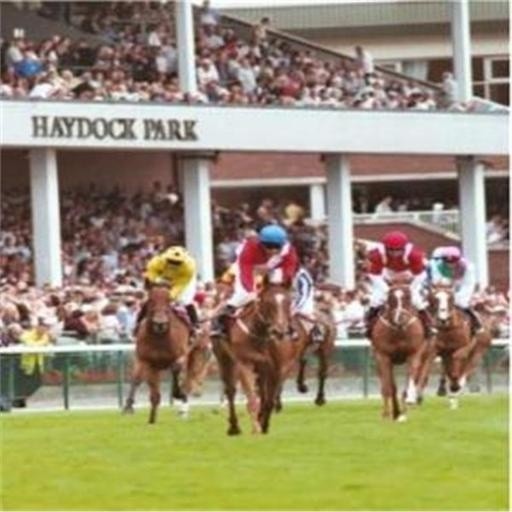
xmin=428 ymin=246 xmax=481 ymax=329
xmin=274 ymin=258 xmax=316 ymax=336
xmin=0 ymin=182 xmax=509 ymax=344
xmin=362 ymin=231 xmax=440 ymax=338
xmin=0 ymin=0 xmax=485 ymax=111
xmin=134 ymin=246 xmax=201 ymax=334
xmin=210 ymin=225 xmax=298 ymax=334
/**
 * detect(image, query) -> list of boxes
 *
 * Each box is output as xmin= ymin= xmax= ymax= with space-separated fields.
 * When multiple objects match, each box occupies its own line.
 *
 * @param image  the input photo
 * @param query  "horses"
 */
xmin=288 ymin=296 xmax=339 ymax=407
xmin=423 ymin=273 xmax=493 ymax=412
xmin=365 ymin=275 xmax=430 ymax=424
xmin=207 ymin=270 xmax=295 ymax=437
xmin=119 ymin=272 xmax=202 ymax=426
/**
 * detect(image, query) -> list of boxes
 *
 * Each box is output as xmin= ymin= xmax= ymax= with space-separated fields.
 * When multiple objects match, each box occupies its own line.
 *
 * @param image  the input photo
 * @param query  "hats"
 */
xmin=258 ymin=224 xmax=289 ymax=249
xmin=165 ymin=245 xmax=189 ymax=264
xmin=382 ymin=230 xmax=408 ymax=250
xmin=440 ymin=246 xmax=461 ymax=263
